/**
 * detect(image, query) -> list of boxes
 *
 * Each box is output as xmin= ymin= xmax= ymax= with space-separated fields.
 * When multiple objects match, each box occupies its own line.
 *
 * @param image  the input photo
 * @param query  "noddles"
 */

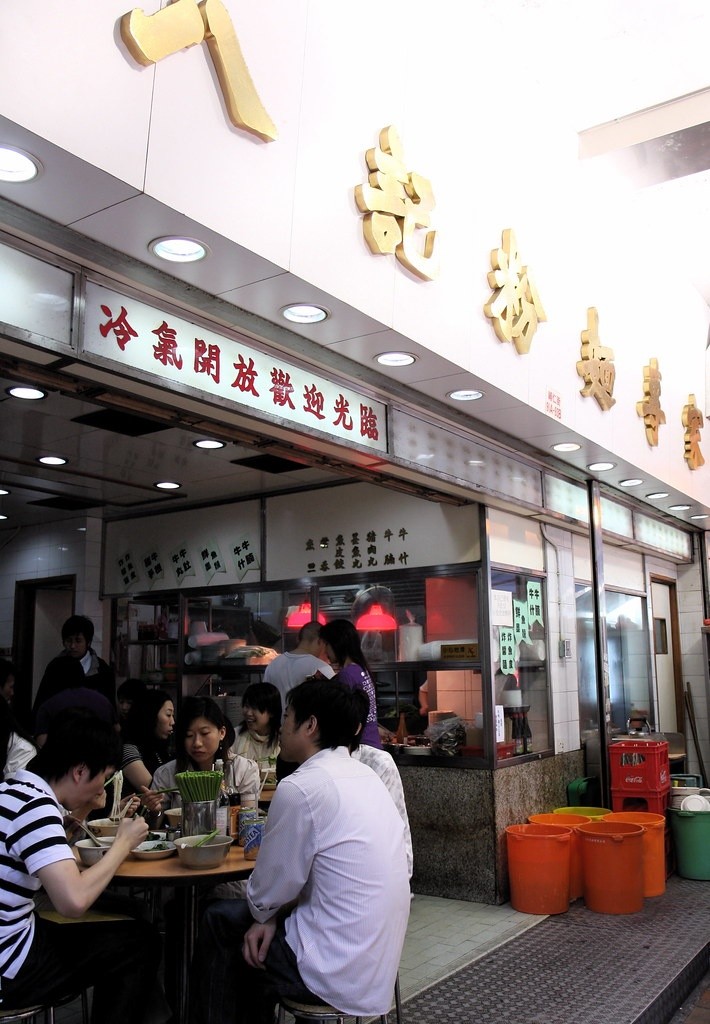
xmin=110 ymin=769 xmax=124 ymax=826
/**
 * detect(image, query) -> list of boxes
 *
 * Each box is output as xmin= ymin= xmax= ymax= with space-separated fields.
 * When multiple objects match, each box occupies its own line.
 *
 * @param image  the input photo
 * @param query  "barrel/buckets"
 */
xmin=504 ymin=807 xmax=710 ymax=914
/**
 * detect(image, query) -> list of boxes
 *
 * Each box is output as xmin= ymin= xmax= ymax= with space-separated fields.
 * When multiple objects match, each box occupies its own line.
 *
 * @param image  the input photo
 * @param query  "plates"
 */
xmin=680 ymin=794 xmax=710 ymax=811
xmin=129 ymin=840 xmax=176 ymax=859
xmin=260 ymin=783 xmax=276 ymax=790
xmin=195 ymin=694 xmax=246 ymax=729
xmin=260 ymin=768 xmax=276 ymax=781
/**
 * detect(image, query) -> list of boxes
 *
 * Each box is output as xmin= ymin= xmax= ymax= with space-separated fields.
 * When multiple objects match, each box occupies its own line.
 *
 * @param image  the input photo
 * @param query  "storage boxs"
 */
xmin=608 ymin=740 xmax=673 ymax=880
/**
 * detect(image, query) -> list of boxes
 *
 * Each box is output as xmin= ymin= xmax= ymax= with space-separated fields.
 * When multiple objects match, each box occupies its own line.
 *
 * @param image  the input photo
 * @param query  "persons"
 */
xmin=263 ymin=620 xmax=335 ymax=726
xmin=419 ymin=680 xmax=429 ymax=716
xmin=33 ymin=615 xmax=114 ymax=710
xmin=317 ymin=618 xmax=382 ymax=749
xmin=0 ymin=659 xmax=414 ymax=1024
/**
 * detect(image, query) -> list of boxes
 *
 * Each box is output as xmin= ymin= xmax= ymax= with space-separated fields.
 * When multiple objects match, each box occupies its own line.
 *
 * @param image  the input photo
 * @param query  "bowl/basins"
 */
xmin=397 ymin=624 xmax=479 ymax=662
xmin=499 ymin=638 xmax=546 ymax=707
xmin=164 ymin=807 xmax=183 ymax=829
xmin=88 ymin=817 xmax=131 ymax=835
xmin=402 ymin=746 xmax=431 ymax=756
xmin=75 ymin=836 xmax=118 ymax=867
xmin=173 ymin=832 xmax=234 ymax=870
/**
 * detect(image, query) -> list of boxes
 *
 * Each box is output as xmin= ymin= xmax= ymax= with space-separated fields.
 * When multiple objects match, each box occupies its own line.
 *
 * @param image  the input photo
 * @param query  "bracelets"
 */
xmin=149 ymin=811 xmax=161 ymax=819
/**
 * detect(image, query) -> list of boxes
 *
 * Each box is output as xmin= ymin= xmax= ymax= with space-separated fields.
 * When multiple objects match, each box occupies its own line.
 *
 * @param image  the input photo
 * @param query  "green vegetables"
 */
xmin=145 ymin=831 xmax=170 ymax=852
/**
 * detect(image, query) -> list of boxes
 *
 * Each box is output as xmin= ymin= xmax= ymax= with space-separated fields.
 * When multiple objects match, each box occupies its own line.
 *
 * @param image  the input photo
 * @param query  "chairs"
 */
xmin=277 ymin=971 xmax=401 ymax=1024
xmin=0 ymin=987 xmax=88 ymax=1024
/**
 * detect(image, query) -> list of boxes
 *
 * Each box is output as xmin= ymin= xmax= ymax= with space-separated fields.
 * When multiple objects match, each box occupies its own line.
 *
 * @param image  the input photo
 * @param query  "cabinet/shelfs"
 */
xmin=120 ymin=597 xmax=251 ymax=702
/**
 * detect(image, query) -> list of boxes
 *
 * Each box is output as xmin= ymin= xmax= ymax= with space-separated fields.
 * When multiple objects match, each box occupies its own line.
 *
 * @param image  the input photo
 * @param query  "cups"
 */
xmin=184 ymin=621 xmax=232 ymax=665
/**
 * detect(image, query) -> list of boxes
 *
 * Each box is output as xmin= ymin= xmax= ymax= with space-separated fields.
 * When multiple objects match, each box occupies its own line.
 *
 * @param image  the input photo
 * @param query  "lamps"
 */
xmin=287 ymin=589 xmax=326 ymax=626
xmin=356 ymin=582 xmax=397 ymax=629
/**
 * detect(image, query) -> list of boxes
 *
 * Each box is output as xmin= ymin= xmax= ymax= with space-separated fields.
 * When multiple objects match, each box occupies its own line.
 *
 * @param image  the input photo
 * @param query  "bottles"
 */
xmin=506 ymin=711 xmax=534 ymax=757
xmin=617 ymin=743 xmax=666 ymax=766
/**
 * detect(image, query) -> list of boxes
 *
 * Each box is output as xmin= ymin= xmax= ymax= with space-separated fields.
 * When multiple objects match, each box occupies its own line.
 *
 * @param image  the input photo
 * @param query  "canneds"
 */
xmin=238 ymin=807 xmax=266 ymax=861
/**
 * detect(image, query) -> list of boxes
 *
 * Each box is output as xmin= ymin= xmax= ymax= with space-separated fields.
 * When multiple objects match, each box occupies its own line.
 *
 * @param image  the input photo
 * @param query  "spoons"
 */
xmin=109 ymin=798 xmax=135 ymax=822
xmin=76 ymin=822 xmax=114 ymax=848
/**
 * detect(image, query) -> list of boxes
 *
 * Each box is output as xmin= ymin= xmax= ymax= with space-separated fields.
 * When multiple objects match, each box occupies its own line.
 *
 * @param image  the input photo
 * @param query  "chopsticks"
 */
xmin=176 ymin=770 xmax=224 ymax=803
xmin=136 ymin=787 xmax=179 ymax=798
xmin=132 ymin=813 xmax=137 ymax=820
xmin=104 ymin=769 xmax=123 ymax=788
xmin=194 ymin=828 xmax=222 ymax=849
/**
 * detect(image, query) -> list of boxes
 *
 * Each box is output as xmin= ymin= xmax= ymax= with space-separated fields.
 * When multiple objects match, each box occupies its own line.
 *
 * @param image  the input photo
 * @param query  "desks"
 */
xmin=96 ymin=846 xmax=254 ymax=1024
xmin=257 ymin=789 xmax=276 ymax=809
